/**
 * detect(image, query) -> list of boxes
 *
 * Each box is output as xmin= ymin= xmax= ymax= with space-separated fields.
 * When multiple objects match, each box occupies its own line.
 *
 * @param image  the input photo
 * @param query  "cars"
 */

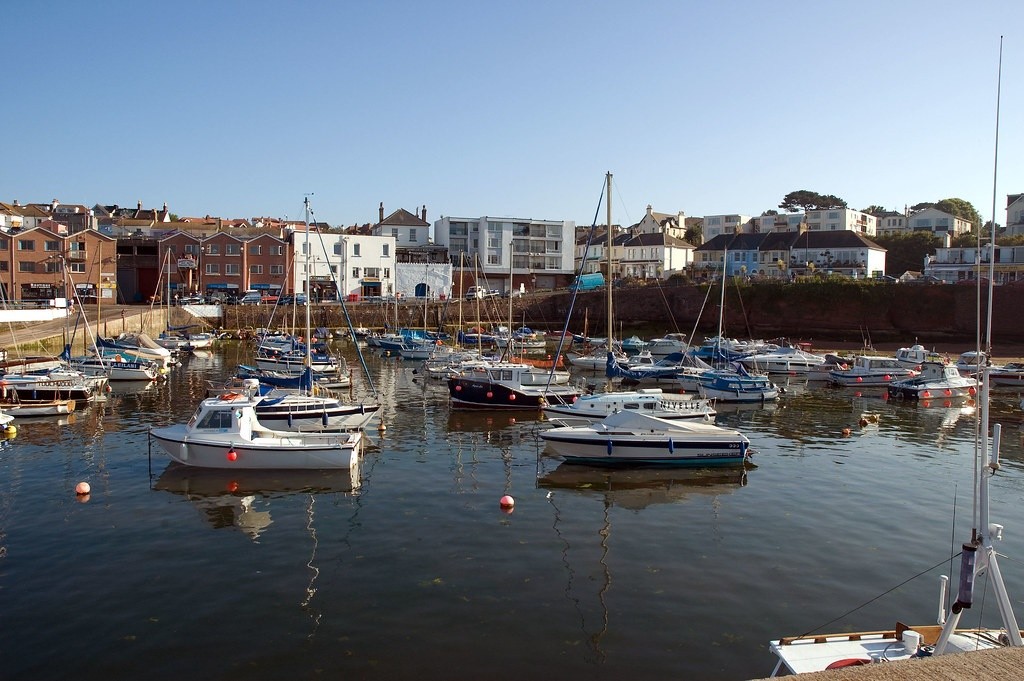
xmin=903 ymin=275 xmax=943 ymax=285
xmin=501 ymin=289 xmax=521 ymax=297
xmin=177 ymin=296 xmax=205 ymax=305
xmin=882 ymin=276 xmax=898 ymax=282
xmin=957 ymin=277 xmax=995 ymax=287
xmin=238 ymin=292 xmax=261 ymax=306
xmin=692 ymin=277 xmax=708 ymax=284
xmin=211 ymin=292 xmax=234 ymax=304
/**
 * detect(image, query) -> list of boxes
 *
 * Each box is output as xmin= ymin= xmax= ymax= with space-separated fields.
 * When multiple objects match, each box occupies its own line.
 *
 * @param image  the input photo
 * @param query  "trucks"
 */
xmin=467 ymin=286 xmax=499 ymax=300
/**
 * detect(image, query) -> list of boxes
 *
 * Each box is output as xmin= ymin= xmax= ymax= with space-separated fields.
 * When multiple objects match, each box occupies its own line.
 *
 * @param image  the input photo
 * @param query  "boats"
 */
xmin=147 ymin=393 xmax=362 ymax=469
xmin=541 ymin=170 xmax=716 ymax=427
xmin=366 ymin=254 xmax=1024 ymax=405
xmin=216 ymin=249 xmax=371 ymax=392
xmin=540 ymin=409 xmax=760 ymax=468
xmin=76 ymin=355 xmax=159 ymax=380
xmin=0 ymin=242 xmax=214 ymax=445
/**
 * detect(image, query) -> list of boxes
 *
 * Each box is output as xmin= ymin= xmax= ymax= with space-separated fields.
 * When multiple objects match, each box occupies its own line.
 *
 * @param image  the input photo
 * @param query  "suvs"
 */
xmin=279 ymin=293 xmax=306 ymax=305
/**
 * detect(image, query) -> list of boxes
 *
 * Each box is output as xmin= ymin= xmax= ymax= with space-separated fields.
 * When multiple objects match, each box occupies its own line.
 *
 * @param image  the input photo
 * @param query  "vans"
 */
xmin=569 ymin=273 xmax=605 ymax=293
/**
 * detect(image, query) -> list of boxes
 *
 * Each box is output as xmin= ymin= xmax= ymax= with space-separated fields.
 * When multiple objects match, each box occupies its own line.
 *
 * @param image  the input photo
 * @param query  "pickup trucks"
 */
xmin=261 ymin=294 xmax=279 ymax=303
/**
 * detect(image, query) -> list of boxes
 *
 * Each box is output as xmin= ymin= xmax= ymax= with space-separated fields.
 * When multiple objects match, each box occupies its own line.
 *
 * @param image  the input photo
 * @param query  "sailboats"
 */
xmin=205 ymin=197 xmax=380 ymax=430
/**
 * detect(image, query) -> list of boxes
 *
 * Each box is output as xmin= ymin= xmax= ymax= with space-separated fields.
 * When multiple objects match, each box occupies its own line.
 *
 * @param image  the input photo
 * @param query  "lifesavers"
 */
xmin=219 ymin=392 xmax=245 ymax=401
